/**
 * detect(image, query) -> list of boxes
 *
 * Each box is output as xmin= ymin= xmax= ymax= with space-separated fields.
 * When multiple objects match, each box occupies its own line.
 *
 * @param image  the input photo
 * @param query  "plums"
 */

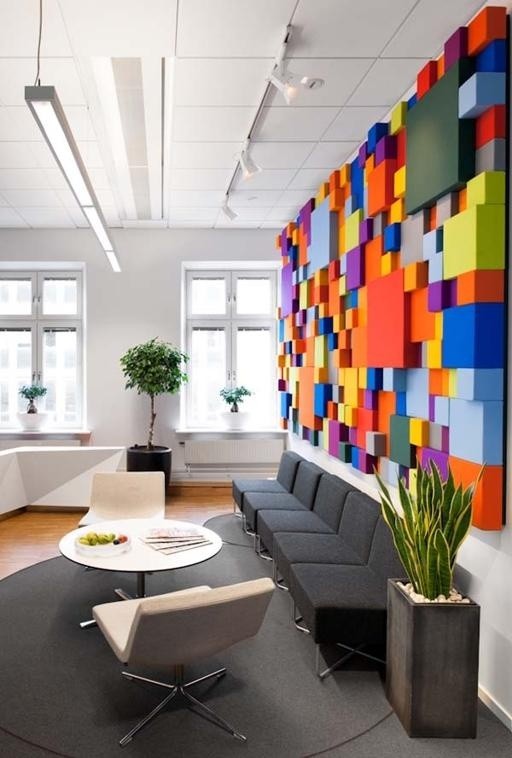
xmin=113 ymin=540 xmax=119 ymax=544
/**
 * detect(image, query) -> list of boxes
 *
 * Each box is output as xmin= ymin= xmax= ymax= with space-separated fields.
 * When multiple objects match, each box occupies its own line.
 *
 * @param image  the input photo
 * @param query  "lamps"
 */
xmin=218 ymin=23 xmax=297 ymax=227
xmin=24 ymin=85 xmax=121 ymax=275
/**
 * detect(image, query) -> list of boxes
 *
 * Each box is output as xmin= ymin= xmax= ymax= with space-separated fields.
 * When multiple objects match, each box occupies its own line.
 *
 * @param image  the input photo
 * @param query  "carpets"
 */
xmin=0 ymin=512 xmax=396 ymax=758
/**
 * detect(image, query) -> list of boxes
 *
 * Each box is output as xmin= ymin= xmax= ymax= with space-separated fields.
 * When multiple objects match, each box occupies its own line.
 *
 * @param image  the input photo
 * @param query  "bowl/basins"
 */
xmin=77 ymin=536 xmax=131 ymax=558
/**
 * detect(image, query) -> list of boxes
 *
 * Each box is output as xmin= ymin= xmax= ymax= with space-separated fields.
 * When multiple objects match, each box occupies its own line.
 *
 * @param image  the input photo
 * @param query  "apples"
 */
xmin=118 ymin=535 xmax=127 ymax=543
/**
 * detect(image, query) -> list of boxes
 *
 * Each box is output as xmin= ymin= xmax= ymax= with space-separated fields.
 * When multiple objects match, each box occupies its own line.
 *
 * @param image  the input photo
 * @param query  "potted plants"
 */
xmin=221 ymin=384 xmax=251 ymax=430
xmin=14 ymin=385 xmax=50 ymax=433
xmin=119 ymin=336 xmax=190 ymax=491
xmin=371 ymin=453 xmax=486 ymax=740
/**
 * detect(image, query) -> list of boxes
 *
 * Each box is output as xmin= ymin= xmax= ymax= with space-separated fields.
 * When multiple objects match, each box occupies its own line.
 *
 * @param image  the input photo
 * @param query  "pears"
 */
xmin=79 ymin=532 xmax=115 ymax=546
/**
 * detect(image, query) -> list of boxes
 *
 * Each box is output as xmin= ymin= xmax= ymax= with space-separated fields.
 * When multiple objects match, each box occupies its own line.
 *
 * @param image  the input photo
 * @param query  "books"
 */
xmin=138 ymin=526 xmax=212 ymax=556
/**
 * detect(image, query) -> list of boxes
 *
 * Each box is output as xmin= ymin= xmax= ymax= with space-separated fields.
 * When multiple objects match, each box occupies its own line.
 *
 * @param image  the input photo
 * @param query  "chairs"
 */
xmin=93 ymin=576 xmax=275 ymax=747
xmin=78 ymin=472 xmax=165 ymax=571
